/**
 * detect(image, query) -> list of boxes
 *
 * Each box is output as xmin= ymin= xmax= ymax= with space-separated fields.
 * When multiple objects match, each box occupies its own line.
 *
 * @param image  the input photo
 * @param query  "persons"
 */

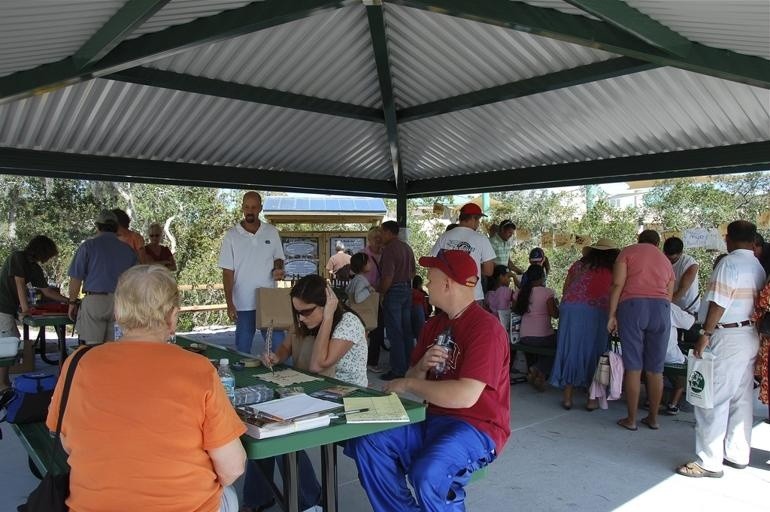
xmin=676 ymin=221 xmax=766 ymax=477
xmin=0 ymin=208 xmax=176 ymax=389
xmin=264 ymin=275 xmax=368 ymax=511
xmin=752 ymin=241 xmax=770 ymax=420
xmin=355 ymin=251 xmax=511 ymax=511
xmin=608 ymin=230 xmax=677 ymax=431
xmin=549 ymin=237 xmax=623 ymax=412
xmin=432 ymin=204 xmax=557 ymax=392
xmin=327 ymin=221 xmax=432 ymax=381
xmin=754 ymin=233 xmax=766 ymax=256
xmin=218 ymin=191 xmax=286 ymax=360
xmin=663 ymin=237 xmax=701 ymax=341
xmin=645 ymin=299 xmax=696 ymax=415
xmin=46 ymin=264 xmax=248 ymax=510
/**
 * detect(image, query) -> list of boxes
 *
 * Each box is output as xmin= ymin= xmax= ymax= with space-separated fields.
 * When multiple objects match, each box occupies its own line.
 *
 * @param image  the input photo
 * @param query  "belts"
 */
xmin=715 ymin=320 xmax=754 ymax=328
xmin=84 ymin=292 xmax=108 ymax=295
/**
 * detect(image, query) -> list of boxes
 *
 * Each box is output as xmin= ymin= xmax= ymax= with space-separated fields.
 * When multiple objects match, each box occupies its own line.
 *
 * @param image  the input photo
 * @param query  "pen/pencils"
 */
xmin=336 ymin=408 xmax=369 ymax=417
xmin=269 ymin=362 xmax=273 ymax=374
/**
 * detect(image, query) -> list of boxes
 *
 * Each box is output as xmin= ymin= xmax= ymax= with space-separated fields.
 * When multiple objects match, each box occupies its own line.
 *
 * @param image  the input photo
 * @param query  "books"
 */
xmin=343 ymin=392 xmax=410 ymax=423
xmin=236 ymin=393 xmax=344 ymax=440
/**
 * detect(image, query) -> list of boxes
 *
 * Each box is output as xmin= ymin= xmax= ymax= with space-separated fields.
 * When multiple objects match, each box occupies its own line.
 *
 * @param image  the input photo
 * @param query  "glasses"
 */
xmin=294 ymin=306 xmax=318 ymax=317
xmin=501 ymin=221 xmax=512 ymax=228
xmin=437 ymin=249 xmax=454 ymax=275
xmin=149 ymin=234 xmax=160 ymax=237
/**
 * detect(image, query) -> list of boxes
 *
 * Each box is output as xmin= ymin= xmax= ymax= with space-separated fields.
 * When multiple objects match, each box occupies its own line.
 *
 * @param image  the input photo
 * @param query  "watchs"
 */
xmin=699 ymin=329 xmax=713 ymax=338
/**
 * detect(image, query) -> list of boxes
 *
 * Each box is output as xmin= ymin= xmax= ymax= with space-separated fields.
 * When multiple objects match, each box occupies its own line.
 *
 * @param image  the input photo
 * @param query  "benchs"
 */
xmin=510 ymin=343 xmax=686 ymax=370
xmin=336 ymin=440 xmax=488 ymax=502
xmin=2 ymin=406 xmax=71 ymax=479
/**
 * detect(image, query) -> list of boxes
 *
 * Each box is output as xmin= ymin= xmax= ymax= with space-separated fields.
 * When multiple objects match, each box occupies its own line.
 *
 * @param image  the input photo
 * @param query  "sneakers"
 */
xmin=677 ymin=463 xmax=723 ymax=477
xmin=723 ymin=459 xmax=747 ymax=469
xmin=644 ymin=401 xmax=663 ymax=410
xmin=368 ymin=366 xmax=383 ymax=373
xmin=667 ymin=402 xmax=681 ymax=414
xmin=382 ymin=371 xmax=404 ymax=379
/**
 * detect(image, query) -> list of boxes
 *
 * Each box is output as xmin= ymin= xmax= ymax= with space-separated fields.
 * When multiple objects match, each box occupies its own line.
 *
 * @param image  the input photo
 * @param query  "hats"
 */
xmin=419 ymin=250 xmax=479 ymax=287
xmin=582 ymin=240 xmax=622 ymax=257
xmin=94 ymin=208 xmax=118 ymax=224
xmin=529 ymin=248 xmax=545 ymax=262
xmin=460 ymin=203 xmax=488 ymax=217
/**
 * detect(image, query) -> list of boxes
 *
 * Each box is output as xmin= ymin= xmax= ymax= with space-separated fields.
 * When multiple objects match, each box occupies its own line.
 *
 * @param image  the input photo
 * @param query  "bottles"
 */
xmin=218 ymin=358 xmax=235 ymax=409
xmin=429 ymin=326 xmax=453 ymax=378
xmin=29 ymin=287 xmax=36 ymax=304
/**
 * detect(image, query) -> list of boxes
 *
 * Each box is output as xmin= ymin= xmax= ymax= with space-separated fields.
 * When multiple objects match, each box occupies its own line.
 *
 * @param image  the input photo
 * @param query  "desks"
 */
xmin=14 ymin=304 xmax=77 ymax=376
xmin=67 ymin=335 xmax=428 ymax=512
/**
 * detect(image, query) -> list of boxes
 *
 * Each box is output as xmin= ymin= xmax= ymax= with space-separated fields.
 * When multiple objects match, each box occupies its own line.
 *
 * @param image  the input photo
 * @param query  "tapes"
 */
xmin=240 ymin=358 xmax=260 ymax=367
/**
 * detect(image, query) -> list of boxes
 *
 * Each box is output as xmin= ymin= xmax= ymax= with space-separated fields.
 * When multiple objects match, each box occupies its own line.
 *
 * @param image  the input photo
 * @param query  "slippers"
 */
xmin=586 ymin=401 xmax=599 ymax=412
xmin=527 ymin=372 xmax=545 ymax=392
xmin=640 ymin=417 xmax=657 ymax=429
xmin=617 ymin=417 xmax=637 ymax=430
xmin=564 ymin=405 xmax=573 ymax=410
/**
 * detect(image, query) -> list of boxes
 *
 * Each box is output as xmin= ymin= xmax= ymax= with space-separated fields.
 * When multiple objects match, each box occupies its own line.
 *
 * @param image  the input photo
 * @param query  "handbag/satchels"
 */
xmin=0 ymin=373 xmax=62 ymax=424
xmin=348 ymin=291 xmax=379 ymax=331
xmin=499 ymin=309 xmax=522 ymax=344
xmin=256 ymin=287 xmax=294 ymax=330
xmin=17 ymin=470 xmax=68 ymax=512
xmin=594 ymin=356 xmax=610 ymax=385
